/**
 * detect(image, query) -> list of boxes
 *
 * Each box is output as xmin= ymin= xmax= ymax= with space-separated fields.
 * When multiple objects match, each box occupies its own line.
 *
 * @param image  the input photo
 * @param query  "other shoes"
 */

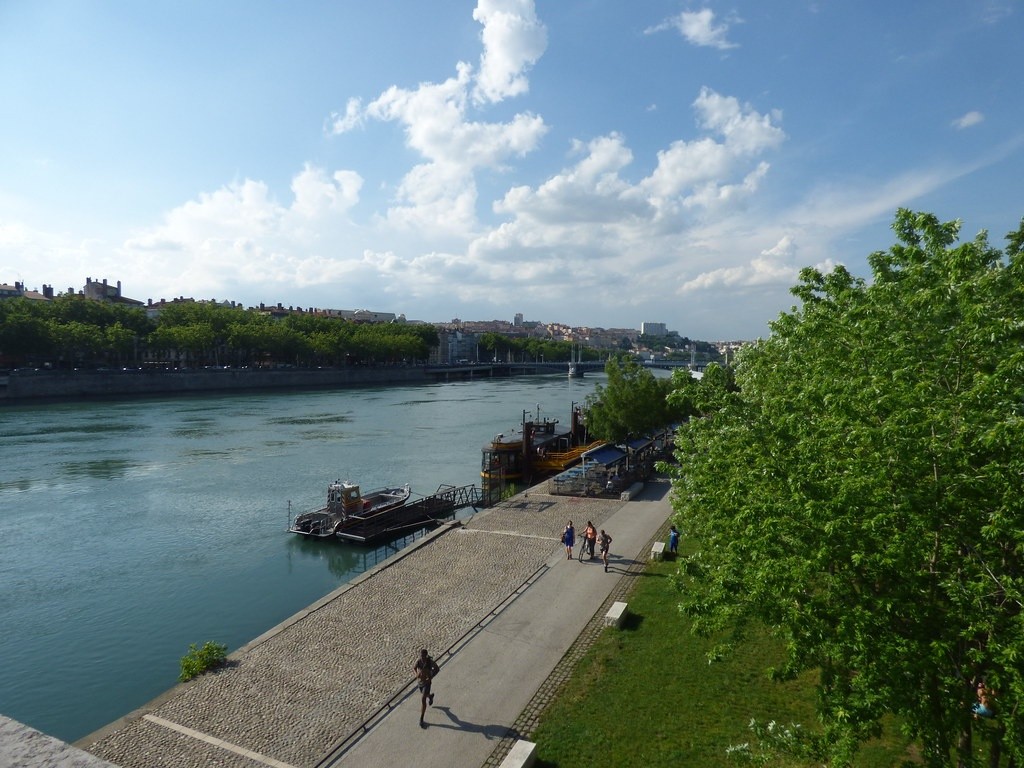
xmin=429 ymin=693 xmax=434 ymax=705
xmin=419 ymin=718 xmax=423 ymax=726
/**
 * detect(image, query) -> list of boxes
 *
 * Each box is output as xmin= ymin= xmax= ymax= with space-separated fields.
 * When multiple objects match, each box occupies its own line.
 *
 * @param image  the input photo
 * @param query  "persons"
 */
xmin=564 ymin=521 xmax=576 ymax=560
xmin=609 ymin=465 xmax=628 ymax=488
xmin=596 ymin=530 xmax=612 ymax=572
xmin=581 ymin=521 xmax=597 ymax=559
xmin=668 ymin=526 xmax=680 ymax=554
xmin=413 ymin=649 xmax=439 ymax=727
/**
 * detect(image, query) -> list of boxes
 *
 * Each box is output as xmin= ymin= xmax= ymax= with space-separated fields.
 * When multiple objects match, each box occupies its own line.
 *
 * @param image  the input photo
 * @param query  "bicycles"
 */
xmin=577 ymin=534 xmax=591 ymax=562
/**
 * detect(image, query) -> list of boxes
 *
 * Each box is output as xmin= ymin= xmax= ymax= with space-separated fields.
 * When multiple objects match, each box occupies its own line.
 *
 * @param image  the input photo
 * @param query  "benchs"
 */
xmin=650 ymin=541 xmax=666 ymax=561
xmin=498 ymin=739 xmax=537 ymax=768
xmin=605 ymin=602 xmax=629 ymax=628
xmin=621 ymin=482 xmax=644 ymax=500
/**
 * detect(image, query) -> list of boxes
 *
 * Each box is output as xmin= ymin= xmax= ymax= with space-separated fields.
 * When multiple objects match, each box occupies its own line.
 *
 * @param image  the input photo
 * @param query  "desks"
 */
xmin=554 ymin=460 xmax=599 ymax=494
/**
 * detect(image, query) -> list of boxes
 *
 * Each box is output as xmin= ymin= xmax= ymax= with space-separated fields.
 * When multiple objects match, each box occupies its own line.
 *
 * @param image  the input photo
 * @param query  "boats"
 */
xmin=479 ymin=401 xmax=606 ymax=497
xmin=284 ymin=478 xmax=412 ymax=539
xmin=567 ymin=361 xmax=586 ymax=379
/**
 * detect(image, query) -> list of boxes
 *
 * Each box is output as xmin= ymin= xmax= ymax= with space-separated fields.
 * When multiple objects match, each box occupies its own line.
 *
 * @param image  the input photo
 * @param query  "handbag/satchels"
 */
xmin=560 ymin=534 xmax=565 ymax=543
